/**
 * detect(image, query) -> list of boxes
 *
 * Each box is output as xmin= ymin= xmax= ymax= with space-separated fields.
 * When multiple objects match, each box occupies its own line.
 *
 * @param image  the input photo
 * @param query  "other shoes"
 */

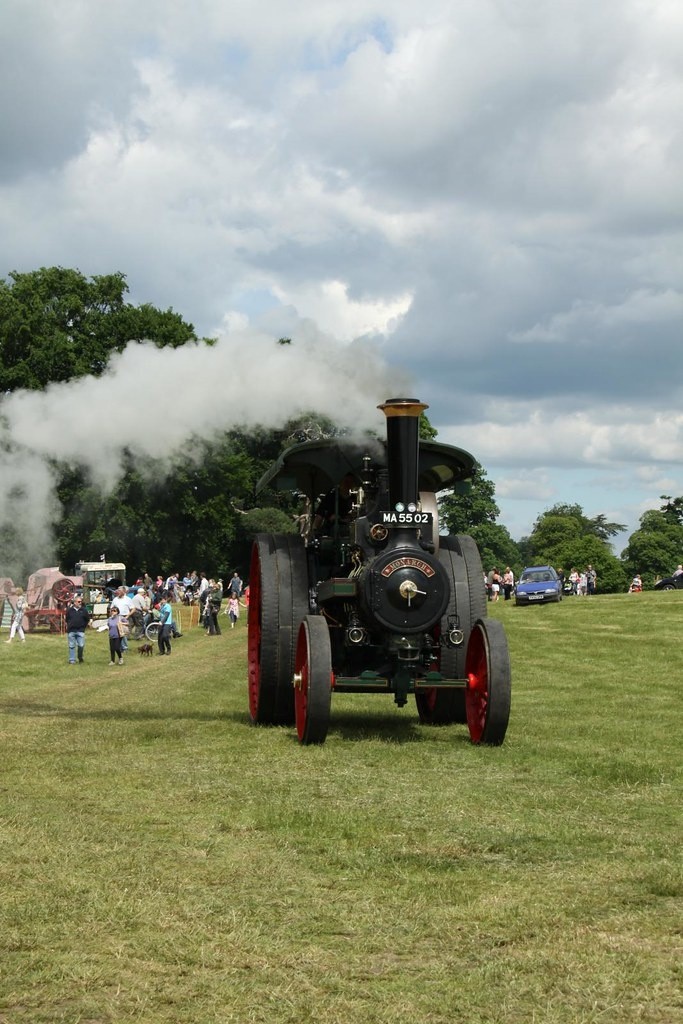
xmin=108 ymin=661 xmax=115 ymax=665
xmin=157 ymin=652 xmax=165 ymax=654
xmin=119 ymin=657 xmax=123 ymax=665
xmin=166 ymin=651 xmax=171 ymax=654
xmin=173 ymin=632 xmax=183 ymax=638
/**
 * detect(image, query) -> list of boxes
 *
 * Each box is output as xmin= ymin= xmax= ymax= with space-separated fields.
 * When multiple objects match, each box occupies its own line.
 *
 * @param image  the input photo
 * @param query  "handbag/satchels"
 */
xmin=116 ymin=616 xmax=130 ymax=637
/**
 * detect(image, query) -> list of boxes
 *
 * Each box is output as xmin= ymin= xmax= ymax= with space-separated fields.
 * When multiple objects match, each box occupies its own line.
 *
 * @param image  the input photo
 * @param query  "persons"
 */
xmin=484 ymin=575 xmax=488 ymax=595
xmin=672 ymin=564 xmax=683 ymax=581
xmin=100 ymin=574 xmax=105 ymax=583
xmin=543 ymin=573 xmax=550 ymax=580
xmin=109 ymin=570 xmax=250 ymax=639
xmin=224 ymin=590 xmax=247 ymax=628
xmin=487 ymin=567 xmax=496 ymax=602
xmin=492 ymin=569 xmax=502 ymax=600
xmin=66 ymin=596 xmax=89 ymax=664
xmin=312 ymin=472 xmax=357 ymax=541
xmin=155 ymin=596 xmax=173 ymax=655
xmin=557 ymin=564 xmax=597 ymax=596
xmin=502 ymin=567 xmax=514 ymax=600
xmin=630 ymin=574 xmax=642 ymax=586
xmin=106 ymin=577 xmax=122 ymax=599
xmin=512 ymin=579 xmax=519 ymax=596
xmin=107 ymin=605 xmax=129 ymax=666
xmin=526 ymin=574 xmax=533 ymax=580
xmin=107 ymin=575 xmax=113 ymax=582
xmin=4 ymin=584 xmax=28 ymax=643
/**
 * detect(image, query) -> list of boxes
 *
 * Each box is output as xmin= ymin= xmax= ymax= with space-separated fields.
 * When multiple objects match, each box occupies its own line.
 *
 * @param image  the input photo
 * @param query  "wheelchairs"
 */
xmin=143 ymin=610 xmax=162 ymax=642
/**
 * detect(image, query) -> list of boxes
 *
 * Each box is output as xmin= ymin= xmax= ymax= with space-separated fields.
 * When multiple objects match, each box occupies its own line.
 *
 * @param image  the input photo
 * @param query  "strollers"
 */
xmin=563 ymin=579 xmax=577 ymax=596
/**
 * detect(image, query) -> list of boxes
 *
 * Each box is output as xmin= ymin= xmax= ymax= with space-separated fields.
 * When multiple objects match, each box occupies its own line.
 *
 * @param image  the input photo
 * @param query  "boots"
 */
xmin=231 ymin=623 xmax=234 ymax=628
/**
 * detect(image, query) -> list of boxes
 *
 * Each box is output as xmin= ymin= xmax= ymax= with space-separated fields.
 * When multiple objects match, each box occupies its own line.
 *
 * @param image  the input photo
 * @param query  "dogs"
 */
xmin=138 ymin=644 xmax=154 ymax=657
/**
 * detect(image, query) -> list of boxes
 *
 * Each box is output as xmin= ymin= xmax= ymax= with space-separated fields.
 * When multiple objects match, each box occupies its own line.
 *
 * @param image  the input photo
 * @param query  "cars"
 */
xmin=513 ymin=564 xmax=563 ymax=606
xmin=654 ymin=571 xmax=683 ymax=590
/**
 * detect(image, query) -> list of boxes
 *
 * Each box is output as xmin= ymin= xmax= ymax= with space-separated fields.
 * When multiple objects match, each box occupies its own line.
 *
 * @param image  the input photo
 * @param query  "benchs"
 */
xmin=317 ymin=536 xmax=348 ymax=563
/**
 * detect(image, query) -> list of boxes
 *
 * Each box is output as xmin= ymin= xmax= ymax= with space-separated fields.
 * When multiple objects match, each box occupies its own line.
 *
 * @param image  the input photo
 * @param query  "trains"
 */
xmin=247 ymin=396 xmax=513 ymax=747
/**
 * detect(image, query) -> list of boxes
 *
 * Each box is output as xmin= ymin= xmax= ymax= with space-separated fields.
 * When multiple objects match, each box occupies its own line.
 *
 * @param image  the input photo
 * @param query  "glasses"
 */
xmin=75 ymin=601 xmax=82 ymax=603
xmin=111 ymin=609 xmax=116 ymax=612
